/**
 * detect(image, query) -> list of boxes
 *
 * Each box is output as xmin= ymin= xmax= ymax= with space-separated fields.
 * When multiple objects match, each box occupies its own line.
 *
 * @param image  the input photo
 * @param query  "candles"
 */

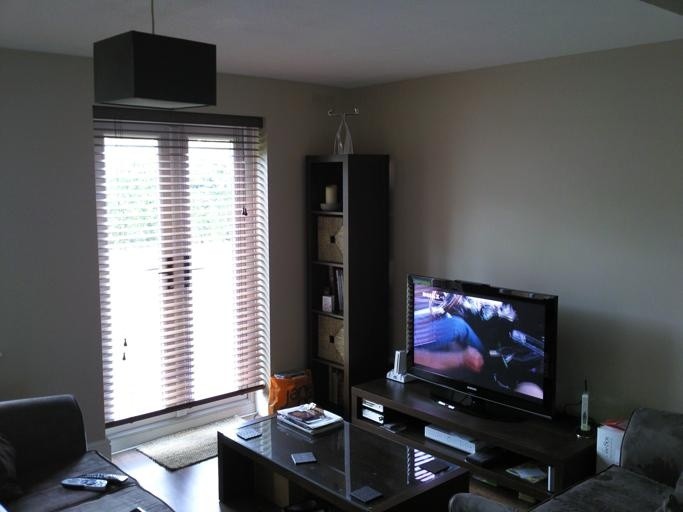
xmin=325 ymin=184 xmax=337 ymax=204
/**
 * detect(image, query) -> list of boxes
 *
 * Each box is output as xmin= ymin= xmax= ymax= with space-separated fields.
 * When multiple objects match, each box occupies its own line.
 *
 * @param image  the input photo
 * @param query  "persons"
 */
xmin=414 ymin=283 xmax=487 ymax=372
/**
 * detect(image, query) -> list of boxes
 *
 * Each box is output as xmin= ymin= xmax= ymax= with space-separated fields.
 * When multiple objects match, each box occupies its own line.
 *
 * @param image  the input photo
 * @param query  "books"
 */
xmin=277 ymin=366 xmax=343 ymax=438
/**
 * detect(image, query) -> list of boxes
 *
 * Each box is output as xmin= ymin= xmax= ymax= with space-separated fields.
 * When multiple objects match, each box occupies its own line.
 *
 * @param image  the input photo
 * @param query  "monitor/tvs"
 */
xmin=406 ymin=273 xmax=558 ymax=420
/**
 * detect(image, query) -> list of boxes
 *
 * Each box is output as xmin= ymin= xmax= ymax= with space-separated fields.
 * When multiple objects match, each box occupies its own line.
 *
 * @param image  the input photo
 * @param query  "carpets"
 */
xmin=134 ymin=414 xmax=249 ymax=472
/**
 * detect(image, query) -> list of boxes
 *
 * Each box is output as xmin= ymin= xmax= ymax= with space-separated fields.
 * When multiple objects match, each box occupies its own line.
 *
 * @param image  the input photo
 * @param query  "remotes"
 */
xmin=61 ymin=477 xmax=108 ymax=492
xmin=78 ymin=472 xmax=128 ymax=485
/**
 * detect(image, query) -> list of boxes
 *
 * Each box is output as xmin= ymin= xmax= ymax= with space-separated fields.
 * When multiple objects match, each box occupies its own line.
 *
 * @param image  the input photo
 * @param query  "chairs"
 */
xmin=0 ymin=393 xmax=176 ymax=512
xmin=449 ymin=405 xmax=683 ymax=512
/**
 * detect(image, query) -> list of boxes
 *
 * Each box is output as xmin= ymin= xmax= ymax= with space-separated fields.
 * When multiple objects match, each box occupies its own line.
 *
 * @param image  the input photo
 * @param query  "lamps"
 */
xmin=92 ymin=0 xmax=218 ymax=109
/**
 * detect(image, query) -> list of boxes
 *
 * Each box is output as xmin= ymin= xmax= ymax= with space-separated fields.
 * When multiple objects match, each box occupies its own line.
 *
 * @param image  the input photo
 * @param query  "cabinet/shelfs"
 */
xmin=306 ymin=153 xmax=390 ymax=422
xmin=351 ymin=377 xmax=598 ymax=503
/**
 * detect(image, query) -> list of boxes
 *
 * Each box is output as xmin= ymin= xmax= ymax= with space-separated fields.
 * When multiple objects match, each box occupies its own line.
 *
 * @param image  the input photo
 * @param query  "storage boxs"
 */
xmin=595 ymin=416 xmax=630 ymax=473
